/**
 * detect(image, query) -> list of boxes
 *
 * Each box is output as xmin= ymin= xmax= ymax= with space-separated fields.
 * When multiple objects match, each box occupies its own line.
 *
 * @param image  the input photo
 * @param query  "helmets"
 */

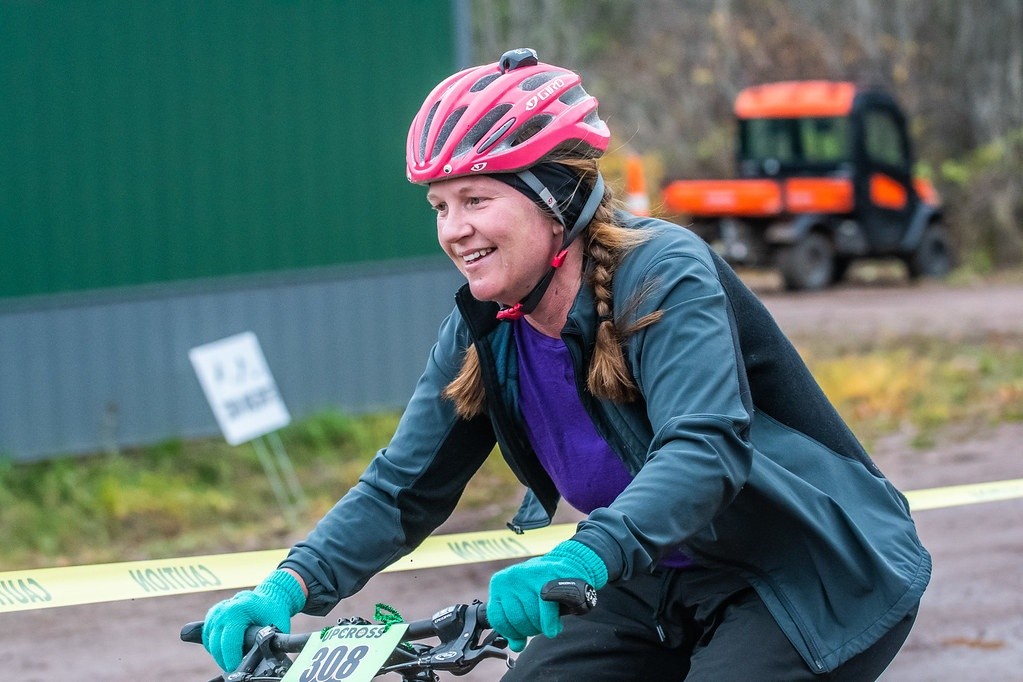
xmin=405 ymin=48 xmax=611 ymax=188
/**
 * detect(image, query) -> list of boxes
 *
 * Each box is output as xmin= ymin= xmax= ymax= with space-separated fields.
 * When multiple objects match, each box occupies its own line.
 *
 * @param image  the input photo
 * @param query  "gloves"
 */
xmin=486 ymin=541 xmax=608 ymax=653
xmin=201 ymin=570 xmax=305 ymax=675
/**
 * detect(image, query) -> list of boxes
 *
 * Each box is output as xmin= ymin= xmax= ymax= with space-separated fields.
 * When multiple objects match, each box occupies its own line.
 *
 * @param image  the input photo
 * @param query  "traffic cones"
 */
xmin=620 ymin=155 xmax=652 ymax=220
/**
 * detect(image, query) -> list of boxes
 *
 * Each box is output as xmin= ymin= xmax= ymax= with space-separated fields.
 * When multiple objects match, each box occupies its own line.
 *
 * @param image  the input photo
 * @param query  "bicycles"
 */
xmin=179 ymin=577 xmax=597 ymax=682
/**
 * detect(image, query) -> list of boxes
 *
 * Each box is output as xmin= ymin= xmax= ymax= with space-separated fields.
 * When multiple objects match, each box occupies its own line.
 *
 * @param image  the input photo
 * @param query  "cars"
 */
xmin=663 ymin=81 xmax=964 ymax=296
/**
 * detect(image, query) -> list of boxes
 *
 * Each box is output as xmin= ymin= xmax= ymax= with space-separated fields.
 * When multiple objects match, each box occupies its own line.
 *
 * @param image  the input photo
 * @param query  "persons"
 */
xmin=205 ymin=52 xmax=933 ymax=682
